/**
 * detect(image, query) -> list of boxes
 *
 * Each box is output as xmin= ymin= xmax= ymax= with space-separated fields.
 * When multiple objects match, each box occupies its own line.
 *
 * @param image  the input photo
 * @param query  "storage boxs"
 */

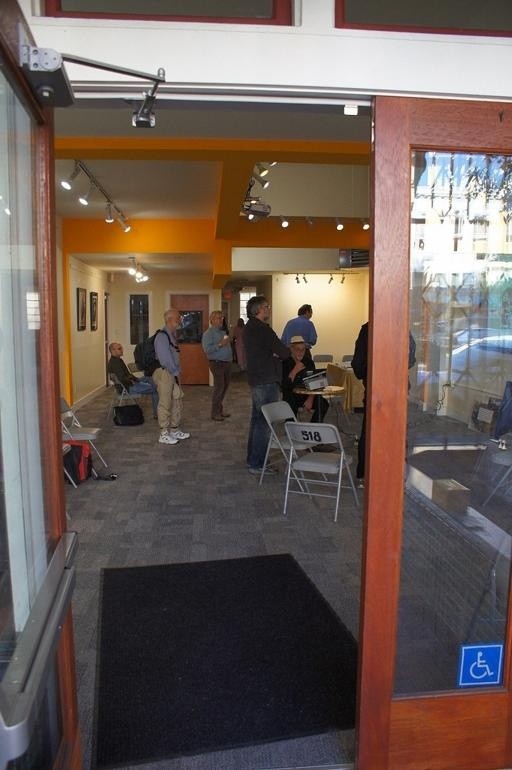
xmin=431 ymin=478 xmax=471 ymax=514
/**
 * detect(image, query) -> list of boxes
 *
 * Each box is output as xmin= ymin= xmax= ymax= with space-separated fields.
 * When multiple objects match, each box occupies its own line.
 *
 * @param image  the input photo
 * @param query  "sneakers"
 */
xmin=221 ymin=413 xmax=230 ymax=417
xmin=248 ymin=465 xmax=280 ymax=476
xmin=170 ymin=430 xmax=190 ymax=439
xmin=356 ymin=479 xmax=366 ymax=489
xmin=211 ymin=416 xmax=224 ymax=421
xmin=159 ymin=432 xmax=179 ymax=445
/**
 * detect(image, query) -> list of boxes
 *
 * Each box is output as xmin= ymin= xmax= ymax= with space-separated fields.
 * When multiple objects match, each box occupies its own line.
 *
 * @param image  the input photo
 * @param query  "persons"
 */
xmin=281 ymin=303 xmax=318 ymax=349
xmin=280 ymin=334 xmax=337 ymax=451
xmin=350 ymin=318 xmax=418 ymax=492
xmin=107 ymin=342 xmax=160 ymax=421
xmin=150 ymin=309 xmax=192 ymax=445
xmin=231 ymin=316 xmax=249 ymax=371
xmin=241 ymin=295 xmax=291 ymax=477
xmin=201 ymin=310 xmax=235 ymax=421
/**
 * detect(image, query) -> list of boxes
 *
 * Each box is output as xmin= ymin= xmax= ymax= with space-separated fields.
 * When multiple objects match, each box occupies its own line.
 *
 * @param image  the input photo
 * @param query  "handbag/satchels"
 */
xmin=113 ymin=404 xmax=144 ymax=426
xmin=63 ymin=441 xmax=92 ymax=484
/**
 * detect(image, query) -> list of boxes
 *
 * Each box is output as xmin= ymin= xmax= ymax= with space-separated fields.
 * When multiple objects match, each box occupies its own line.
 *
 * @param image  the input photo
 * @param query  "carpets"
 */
xmin=88 ymin=552 xmax=358 ymax=769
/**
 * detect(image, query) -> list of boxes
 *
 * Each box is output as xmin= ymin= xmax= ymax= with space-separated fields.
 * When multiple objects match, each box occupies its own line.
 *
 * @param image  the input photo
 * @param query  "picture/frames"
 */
xmin=76 ymin=287 xmax=87 ymax=331
xmin=90 ymin=291 xmax=98 ymax=331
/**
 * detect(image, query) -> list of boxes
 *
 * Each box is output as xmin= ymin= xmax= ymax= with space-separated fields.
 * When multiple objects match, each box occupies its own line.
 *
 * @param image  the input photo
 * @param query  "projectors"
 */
xmin=245 ymin=202 xmax=271 ymax=219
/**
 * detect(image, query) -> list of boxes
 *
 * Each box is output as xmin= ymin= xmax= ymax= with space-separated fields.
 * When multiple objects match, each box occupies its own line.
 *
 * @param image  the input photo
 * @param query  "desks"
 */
xmin=325 ymin=361 xmax=366 ymax=414
xmin=292 ymin=385 xmax=346 ymax=454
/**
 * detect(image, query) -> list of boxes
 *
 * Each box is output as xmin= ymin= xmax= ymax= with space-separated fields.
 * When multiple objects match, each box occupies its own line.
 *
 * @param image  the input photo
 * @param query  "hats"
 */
xmin=291 ymin=336 xmax=305 ymax=343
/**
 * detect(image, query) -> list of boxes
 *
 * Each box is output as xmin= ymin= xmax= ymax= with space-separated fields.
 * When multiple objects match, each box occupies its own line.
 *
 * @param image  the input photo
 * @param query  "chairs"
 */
xmin=312 ymin=354 xmax=333 ymax=363
xmin=108 ymin=372 xmax=142 ymax=419
xmin=259 ymin=400 xmax=328 ymax=493
xmin=342 ymin=355 xmax=355 ymax=361
xmin=283 ymin=420 xmax=360 ymax=522
xmin=62 ymin=442 xmax=80 ymax=488
xmin=59 ymin=397 xmax=109 ymax=480
xmin=481 ymin=448 xmax=512 ymax=508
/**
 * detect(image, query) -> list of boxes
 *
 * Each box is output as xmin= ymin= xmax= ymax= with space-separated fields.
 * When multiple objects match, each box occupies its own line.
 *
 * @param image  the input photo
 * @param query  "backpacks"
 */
xmin=133 ymin=330 xmax=173 ymax=377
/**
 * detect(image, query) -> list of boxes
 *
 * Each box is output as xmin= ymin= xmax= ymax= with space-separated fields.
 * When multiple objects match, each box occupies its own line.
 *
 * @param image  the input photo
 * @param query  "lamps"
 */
xmin=294 ymin=272 xmax=347 ymax=285
xmin=278 ymin=212 xmax=372 ymax=232
xmin=241 ymin=161 xmax=279 ymax=221
xmin=126 ymin=259 xmax=150 ymax=283
xmin=59 ymin=161 xmax=131 ymax=233
